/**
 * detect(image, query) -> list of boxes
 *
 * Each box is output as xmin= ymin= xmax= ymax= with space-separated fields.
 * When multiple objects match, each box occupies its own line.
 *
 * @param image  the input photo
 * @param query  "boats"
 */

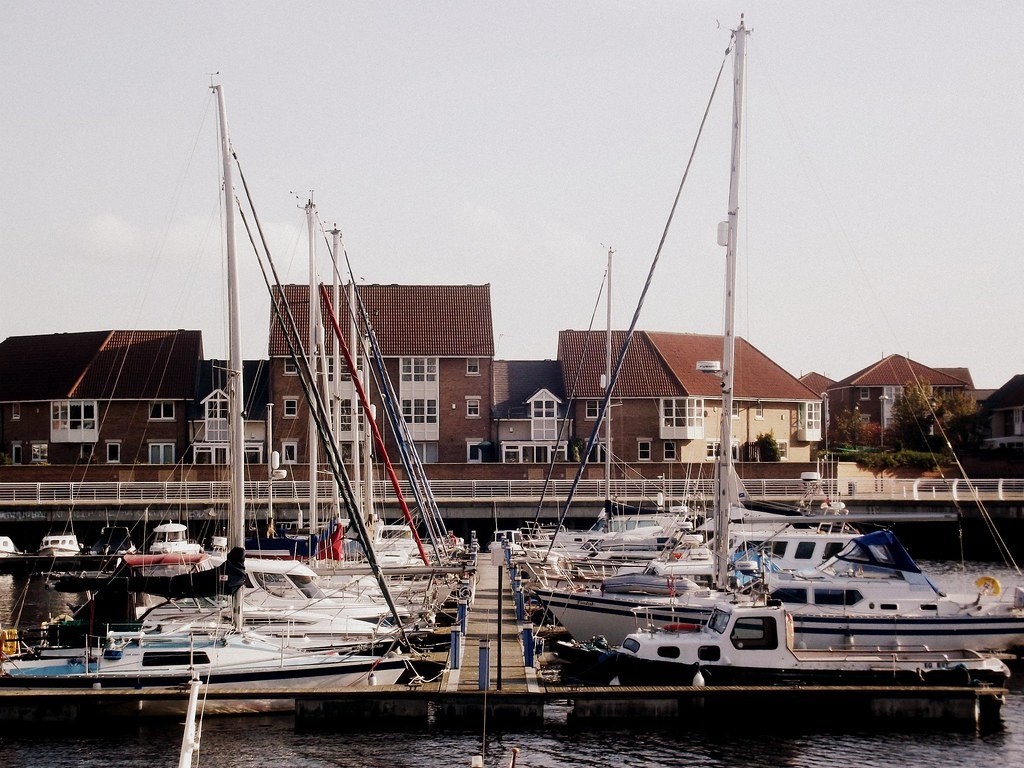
xmin=1 ymin=534 xmax=15 ymax=559
xmin=149 ymin=521 xmax=203 ymax=557
xmin=37 ymin=532 xmax=80 ymax=556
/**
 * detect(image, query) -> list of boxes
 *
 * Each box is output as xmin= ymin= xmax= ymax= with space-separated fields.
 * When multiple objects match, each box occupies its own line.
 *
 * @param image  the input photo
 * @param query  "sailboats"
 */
xmin=1 ymin=73 xmax=465 ymax=723
xmin=513 ymin=14 xmax=1024 ymax=684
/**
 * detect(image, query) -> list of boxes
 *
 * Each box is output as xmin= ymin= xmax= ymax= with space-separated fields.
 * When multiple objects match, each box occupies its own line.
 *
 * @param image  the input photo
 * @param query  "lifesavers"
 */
xmin=444 ymin=535 xmax=457 ymax=546
xmin=662 ymin=624 xmax=703 ymax=632
xmin=975 ymin=577 xmax=1000 ymax=597
